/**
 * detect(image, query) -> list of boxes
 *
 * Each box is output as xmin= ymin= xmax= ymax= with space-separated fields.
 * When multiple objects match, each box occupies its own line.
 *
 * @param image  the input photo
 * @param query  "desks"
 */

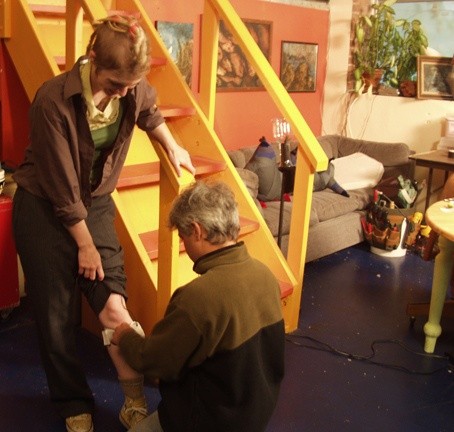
xmin=423 ymin=197 xmax=454 ymax=353
xmin=409 ymin=150 xmax=454 ymax=261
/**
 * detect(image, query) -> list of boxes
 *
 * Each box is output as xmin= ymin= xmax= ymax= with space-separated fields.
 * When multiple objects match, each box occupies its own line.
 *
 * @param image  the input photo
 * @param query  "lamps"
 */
xmin=271 ymin=118 xmax=290 ymax=247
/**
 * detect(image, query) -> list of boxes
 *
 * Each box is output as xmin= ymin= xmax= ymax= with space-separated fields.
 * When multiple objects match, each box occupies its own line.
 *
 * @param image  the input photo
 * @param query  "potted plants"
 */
xmin=351 ymin=0 xmax=428 ymax=97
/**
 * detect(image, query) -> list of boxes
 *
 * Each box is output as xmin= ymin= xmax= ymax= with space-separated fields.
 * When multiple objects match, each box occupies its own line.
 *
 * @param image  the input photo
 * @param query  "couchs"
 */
xmin=228 ymin=134 xmax=416 ymax=263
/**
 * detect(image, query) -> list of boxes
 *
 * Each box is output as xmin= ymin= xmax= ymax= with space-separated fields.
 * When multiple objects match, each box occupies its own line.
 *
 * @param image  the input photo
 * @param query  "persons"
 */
xmin=12 ymin=12 xmax=194 ymax=432
xmin=110 ymin=179 xmax=285 ymax=432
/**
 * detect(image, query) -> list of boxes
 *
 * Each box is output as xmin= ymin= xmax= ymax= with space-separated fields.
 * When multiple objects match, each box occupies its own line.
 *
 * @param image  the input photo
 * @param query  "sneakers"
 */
xmin=119 ymin=403 xmax=147 ymax=429
xmin=64 ymin=414 xmax=94 ymax=432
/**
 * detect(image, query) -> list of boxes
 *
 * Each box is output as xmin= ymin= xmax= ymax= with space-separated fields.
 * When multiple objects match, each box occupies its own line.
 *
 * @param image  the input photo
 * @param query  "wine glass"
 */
xmin=273 ymin=118 xmax=291 ymax=164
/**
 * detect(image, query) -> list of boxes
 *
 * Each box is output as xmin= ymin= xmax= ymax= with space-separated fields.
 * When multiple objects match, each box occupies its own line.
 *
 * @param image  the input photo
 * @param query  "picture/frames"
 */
xmin=280 ymin=40 xmax=319 ymax=93
xmin=198 ymin=14 xmax=273 ymax=92
xmin=416 ymin=55 xmax=454 ymax=99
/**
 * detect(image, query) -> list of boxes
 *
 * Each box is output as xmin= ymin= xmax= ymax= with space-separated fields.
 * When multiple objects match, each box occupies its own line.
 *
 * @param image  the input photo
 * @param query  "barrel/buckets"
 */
xmin=370 ymin=206 xmax=416 ymax=257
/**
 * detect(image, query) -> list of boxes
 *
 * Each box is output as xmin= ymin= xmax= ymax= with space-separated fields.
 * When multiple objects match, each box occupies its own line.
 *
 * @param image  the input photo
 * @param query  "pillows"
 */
xmin=331 ymin=151 xmax=385 ymax=190
xmin=236 ymin=134 xmax=291 ymax=202
